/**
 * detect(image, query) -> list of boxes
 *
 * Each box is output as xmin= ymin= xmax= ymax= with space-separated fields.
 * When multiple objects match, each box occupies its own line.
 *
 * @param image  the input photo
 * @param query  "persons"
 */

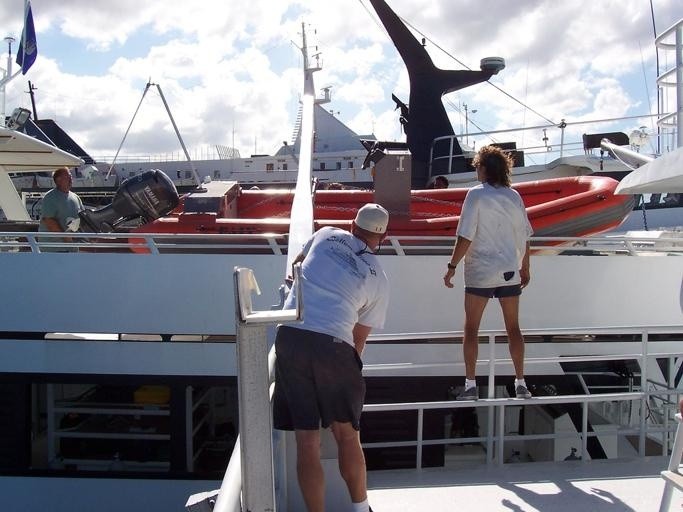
xmin=270 ymin=201 xmax=389 ymax=511
xmin=433 ymin=176 xmax=450 ymax=191
xmin=37 ymin=166 xmax=86 ymax=253
xmin=442 ymin=146 xmax=537 ymax=401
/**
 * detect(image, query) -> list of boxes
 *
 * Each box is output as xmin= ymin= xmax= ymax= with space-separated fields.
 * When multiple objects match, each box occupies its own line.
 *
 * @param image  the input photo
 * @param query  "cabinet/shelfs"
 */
xmin=45 ymin=383 xmax=214 ymax=478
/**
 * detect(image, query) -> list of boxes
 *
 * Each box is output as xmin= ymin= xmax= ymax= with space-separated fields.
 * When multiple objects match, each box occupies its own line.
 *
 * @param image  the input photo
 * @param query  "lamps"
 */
xmin=7 ymin=107 xmax=32 ymax=130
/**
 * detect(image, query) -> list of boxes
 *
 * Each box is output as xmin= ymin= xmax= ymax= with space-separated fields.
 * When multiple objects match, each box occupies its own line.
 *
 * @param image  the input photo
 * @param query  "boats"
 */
xmin=0 ymin=22 xmax=476 ymax=219
xmin=128 ymin=176 xmax=635 ymax=255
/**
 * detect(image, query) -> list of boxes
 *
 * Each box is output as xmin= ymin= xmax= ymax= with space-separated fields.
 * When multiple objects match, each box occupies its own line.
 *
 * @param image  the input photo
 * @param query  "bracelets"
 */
xmin=447 ymin=262 xmax=456 ymax=268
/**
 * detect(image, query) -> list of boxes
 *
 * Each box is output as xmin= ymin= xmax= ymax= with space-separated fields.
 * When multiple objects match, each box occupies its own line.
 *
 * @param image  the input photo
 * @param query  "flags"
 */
xmin=14 ymin=1 xmax=36 ymax=76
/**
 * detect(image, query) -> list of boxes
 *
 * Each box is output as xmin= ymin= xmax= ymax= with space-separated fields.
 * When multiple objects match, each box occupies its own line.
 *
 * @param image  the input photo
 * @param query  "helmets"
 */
xmin=353 ymin=202 xmax=389 ymax=234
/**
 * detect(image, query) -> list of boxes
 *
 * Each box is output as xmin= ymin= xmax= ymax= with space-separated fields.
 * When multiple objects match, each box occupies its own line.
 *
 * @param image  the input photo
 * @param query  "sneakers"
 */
xmin=516 ymin=385 xmax=532 ymax=398
xmin=457 ymin=387 xmax=479 ymax=400
